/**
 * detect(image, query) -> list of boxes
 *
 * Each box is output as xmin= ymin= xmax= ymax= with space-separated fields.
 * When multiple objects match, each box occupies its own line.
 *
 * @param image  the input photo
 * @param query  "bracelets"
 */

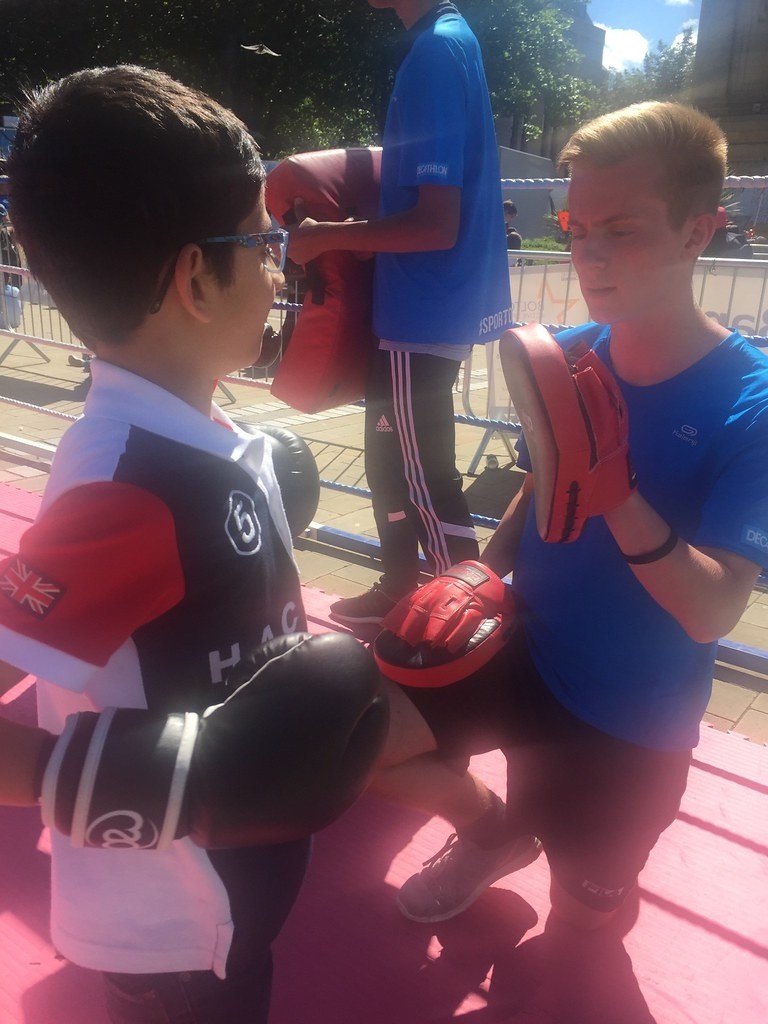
xmin=623 ymin=526 xmax=677 ymax=566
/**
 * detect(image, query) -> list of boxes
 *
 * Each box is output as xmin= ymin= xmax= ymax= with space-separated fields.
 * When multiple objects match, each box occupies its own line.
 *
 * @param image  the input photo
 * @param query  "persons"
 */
xmin=504 ymin=200 xmax=522 ymax=251
xmin=0 ymin=63 xmax=391 ymax=1023
xmin=266 ymin=0 xmax=515 ymax=623
xmin=0 ymin=175 xmax=10 ymax=212
xmin=362 ymin=98 xmax=768 ymax=997
xmin=0 ymin=202 xmax=26 ymax=290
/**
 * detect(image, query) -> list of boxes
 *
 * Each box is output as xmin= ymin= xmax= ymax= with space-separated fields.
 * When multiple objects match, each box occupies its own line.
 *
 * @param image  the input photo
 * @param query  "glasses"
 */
xmin=150 ymin=209 xmax=289 ymax=315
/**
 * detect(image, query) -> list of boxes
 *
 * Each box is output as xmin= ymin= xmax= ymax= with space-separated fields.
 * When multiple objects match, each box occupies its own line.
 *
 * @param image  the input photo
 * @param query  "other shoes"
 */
xmin=329 ymin=581 xmax=420 ymax=623
xmin=397 ymin=823 xmax=542 ymax=922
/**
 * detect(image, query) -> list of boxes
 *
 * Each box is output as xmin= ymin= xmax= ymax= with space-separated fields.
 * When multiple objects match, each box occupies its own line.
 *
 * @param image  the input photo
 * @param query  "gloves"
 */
xmin=244 ymin=420 xmax=321 ymax=540
xmin=39 ymin=631 xmax=389 ymax=854
